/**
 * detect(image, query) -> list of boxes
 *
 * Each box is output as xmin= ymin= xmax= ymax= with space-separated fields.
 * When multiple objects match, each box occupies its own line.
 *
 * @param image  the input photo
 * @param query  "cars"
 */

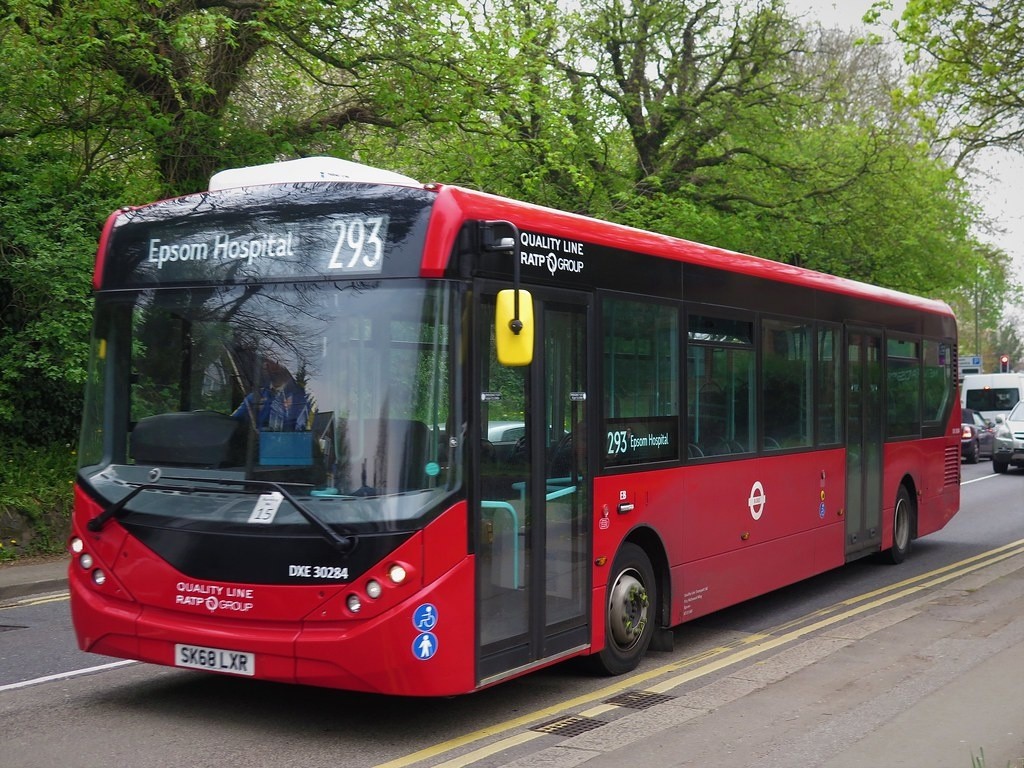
xmin=425 ymin=422 xmax=572 ymax=442
xmin=960 ymin=408 xmax=995 ymax=464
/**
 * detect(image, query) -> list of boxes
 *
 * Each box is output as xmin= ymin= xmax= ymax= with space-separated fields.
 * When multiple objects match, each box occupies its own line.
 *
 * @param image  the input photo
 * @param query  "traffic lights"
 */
xmin=1000 ymin=355 xmax=1009 ymax=373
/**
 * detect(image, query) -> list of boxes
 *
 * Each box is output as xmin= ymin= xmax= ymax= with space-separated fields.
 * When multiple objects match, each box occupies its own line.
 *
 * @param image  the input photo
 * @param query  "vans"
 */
xmin=961 ymin=373 xmax=1024 ymax=425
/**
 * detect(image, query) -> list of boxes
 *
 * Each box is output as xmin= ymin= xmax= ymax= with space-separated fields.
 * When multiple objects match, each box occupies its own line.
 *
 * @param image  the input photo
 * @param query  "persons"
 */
xmin=228 ymin=333 xmax=317 ymax=438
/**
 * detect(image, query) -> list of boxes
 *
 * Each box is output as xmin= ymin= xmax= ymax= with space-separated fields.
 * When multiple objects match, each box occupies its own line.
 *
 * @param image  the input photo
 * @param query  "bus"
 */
xmin=62 ymin=157 xmax=961 ymax=692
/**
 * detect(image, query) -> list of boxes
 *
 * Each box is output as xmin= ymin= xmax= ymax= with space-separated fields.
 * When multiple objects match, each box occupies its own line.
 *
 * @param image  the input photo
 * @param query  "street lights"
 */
xmin=973 ymin=269 xmax=997 ymax=356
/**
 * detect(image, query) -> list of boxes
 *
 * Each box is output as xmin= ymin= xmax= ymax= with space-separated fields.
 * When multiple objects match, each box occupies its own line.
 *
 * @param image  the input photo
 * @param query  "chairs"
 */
xmin=340 ymin=416 xmax=808 ymax=495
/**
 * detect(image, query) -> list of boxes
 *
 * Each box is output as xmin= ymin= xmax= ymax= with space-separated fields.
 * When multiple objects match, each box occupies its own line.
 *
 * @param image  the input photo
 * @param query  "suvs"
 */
xmin=993 ymin=399 xmax=1024 ymax=472
